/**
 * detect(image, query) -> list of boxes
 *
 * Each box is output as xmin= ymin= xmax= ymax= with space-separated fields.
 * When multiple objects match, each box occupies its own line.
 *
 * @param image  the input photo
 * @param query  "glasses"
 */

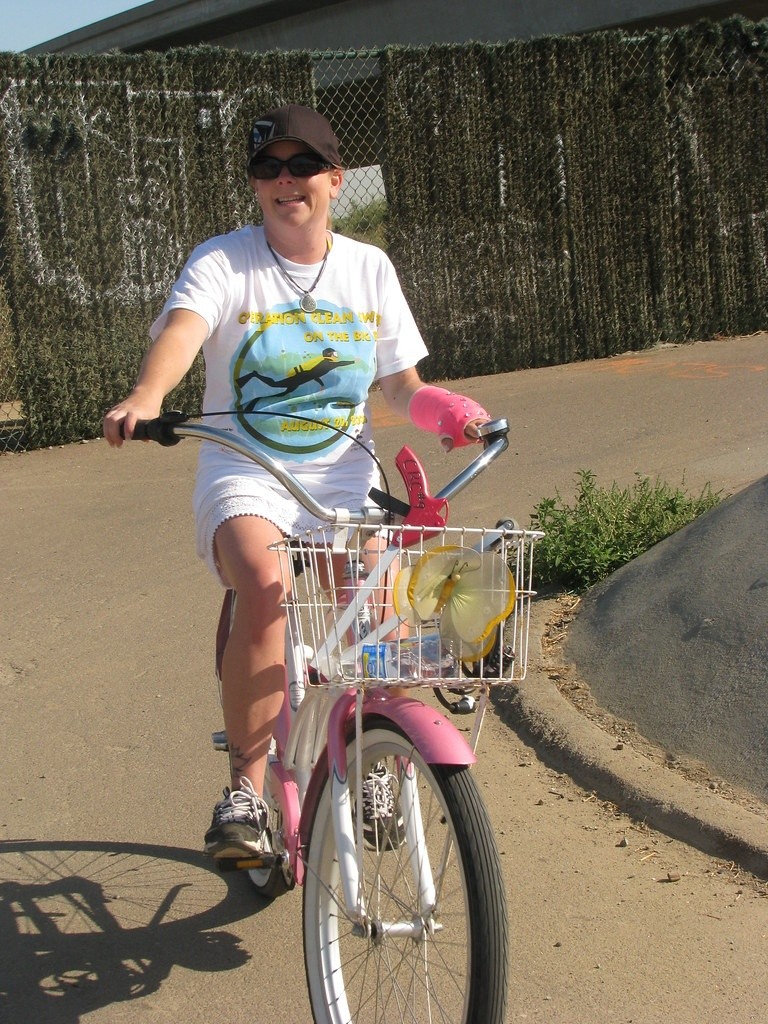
xmin=247 ymin=151 xmax=331 ymax=180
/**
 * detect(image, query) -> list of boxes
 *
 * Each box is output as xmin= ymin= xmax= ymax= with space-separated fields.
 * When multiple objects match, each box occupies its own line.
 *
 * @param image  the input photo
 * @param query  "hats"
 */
xmin=245 ymin=105 xmax=344 ymax=173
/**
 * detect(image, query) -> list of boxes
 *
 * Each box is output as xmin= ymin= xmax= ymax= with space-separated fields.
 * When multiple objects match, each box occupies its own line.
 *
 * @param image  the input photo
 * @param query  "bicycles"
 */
xmin=120 ymin=407 xmax=546 ymax=1024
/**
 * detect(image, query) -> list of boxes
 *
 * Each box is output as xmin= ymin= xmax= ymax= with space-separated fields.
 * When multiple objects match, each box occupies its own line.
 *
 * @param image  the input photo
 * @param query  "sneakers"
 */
xmin=351 ymin=760 xmax=407 ymax=851
xmin=204 ymin=773 xmax=271 ymax=859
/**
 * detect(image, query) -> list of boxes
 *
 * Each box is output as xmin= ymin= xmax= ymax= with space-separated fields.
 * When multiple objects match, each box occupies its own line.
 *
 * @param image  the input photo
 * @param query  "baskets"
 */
xmin=263 ymin=522 xmax=544 ymax=690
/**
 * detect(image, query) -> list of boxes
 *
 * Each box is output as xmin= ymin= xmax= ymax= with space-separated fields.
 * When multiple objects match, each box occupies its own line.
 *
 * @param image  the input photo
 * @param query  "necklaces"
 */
xmin=267 ymin=234 xmax=330 ymax=313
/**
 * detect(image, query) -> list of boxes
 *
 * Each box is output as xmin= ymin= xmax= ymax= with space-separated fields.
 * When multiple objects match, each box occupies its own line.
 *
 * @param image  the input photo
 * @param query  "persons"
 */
xmin=103 ymin=105 xmax=492 ymax=880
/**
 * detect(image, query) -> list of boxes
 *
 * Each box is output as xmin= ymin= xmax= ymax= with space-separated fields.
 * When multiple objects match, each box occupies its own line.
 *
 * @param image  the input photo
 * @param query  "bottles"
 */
xmin=320 ymin=631 xmax=458 ymax=680
